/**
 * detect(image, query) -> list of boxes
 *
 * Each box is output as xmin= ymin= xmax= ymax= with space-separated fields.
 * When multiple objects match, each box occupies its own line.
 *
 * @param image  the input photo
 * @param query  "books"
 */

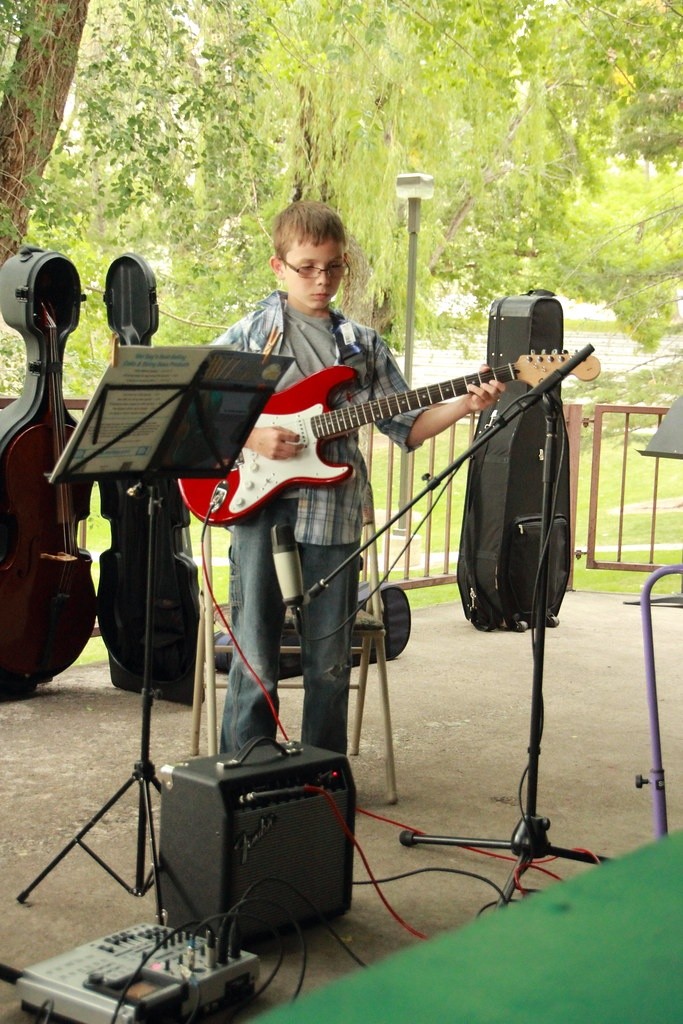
xmin=48 ymin=345 xmax=232 ymax=484
xmin=152 ymin=348 xmax=295 ymax=474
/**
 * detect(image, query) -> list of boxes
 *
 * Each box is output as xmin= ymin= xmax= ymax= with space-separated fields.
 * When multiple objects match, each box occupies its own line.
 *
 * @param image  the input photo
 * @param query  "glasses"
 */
xmin=279 ymin=258 xmax=352 ymax=279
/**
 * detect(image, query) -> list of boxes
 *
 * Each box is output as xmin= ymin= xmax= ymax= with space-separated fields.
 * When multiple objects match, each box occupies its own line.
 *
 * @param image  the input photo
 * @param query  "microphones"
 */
xmin=271 ymin=523 xmax=305 ymax=634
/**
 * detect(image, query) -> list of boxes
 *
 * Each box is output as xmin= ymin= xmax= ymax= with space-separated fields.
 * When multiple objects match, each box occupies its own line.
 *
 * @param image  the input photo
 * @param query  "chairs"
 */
xmin=191 ymin=481 xmax=398 ymax=806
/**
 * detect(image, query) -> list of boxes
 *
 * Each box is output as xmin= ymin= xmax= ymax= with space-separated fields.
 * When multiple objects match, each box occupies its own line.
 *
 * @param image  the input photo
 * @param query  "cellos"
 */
xmin=0 ymin=300 xmax=86 ymax=674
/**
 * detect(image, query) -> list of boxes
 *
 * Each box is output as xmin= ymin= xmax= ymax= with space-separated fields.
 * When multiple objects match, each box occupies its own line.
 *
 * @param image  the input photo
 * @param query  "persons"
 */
xmin=208 ymin=200 xmax=505 ymax=756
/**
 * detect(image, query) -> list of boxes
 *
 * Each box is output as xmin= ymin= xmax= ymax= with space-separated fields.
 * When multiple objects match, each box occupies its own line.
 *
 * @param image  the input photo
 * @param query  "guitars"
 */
xmin=177 ymin=349 xmax=601 ymax=527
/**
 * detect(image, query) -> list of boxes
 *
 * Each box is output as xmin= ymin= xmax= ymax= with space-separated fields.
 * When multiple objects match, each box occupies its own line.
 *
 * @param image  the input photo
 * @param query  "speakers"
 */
xmin=160 ymin=742 xmax=357 ymax=941
